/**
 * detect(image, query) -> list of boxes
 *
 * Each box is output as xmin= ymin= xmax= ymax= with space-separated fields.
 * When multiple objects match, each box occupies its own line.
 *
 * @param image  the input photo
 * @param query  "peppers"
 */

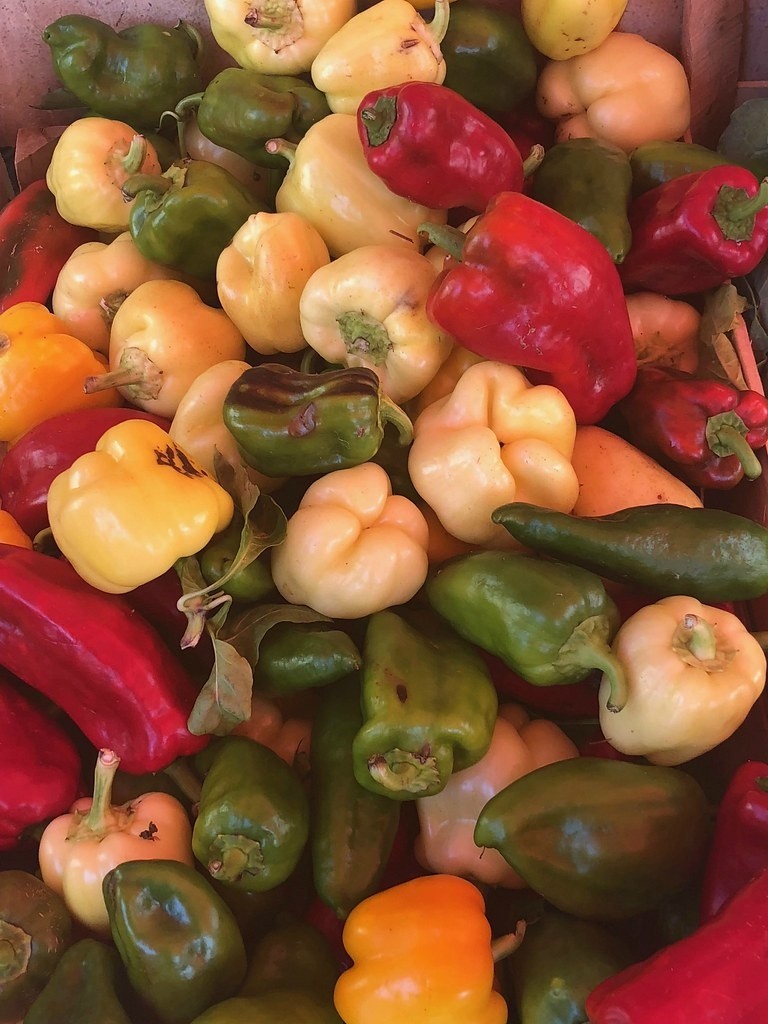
xmin=0 ymin=0 xmax=768 ymax=1024
